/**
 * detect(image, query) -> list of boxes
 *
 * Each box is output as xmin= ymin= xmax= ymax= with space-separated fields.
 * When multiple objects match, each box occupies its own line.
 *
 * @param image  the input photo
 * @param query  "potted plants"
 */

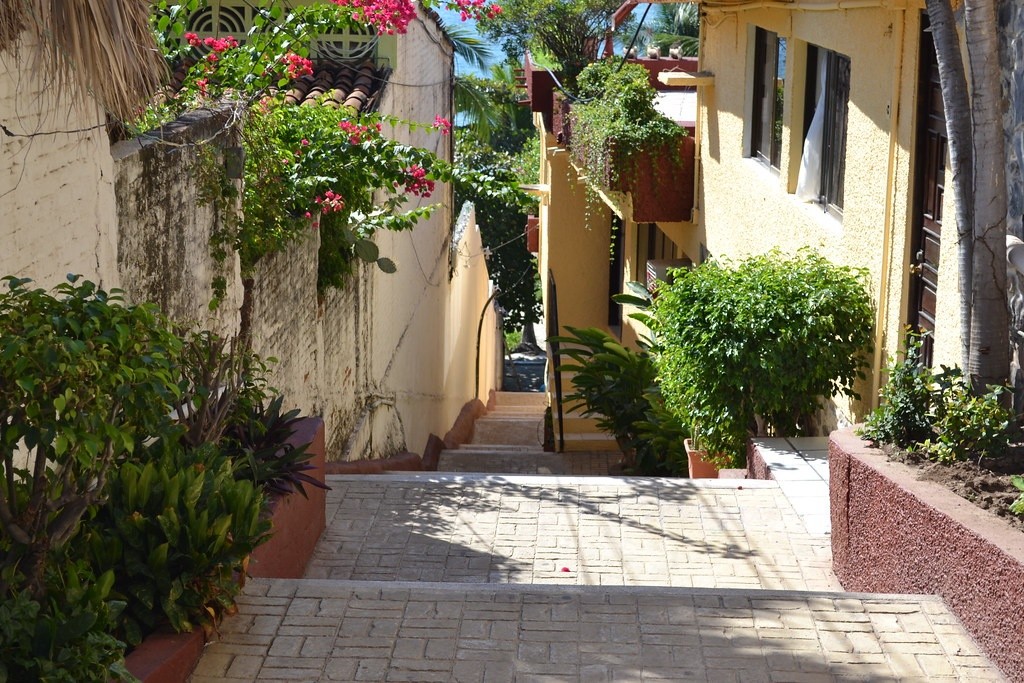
xmin=0 ymin=273 xmax=277 ymax=683
xmin=653 ymin=344 xmax=747 ymax=478
